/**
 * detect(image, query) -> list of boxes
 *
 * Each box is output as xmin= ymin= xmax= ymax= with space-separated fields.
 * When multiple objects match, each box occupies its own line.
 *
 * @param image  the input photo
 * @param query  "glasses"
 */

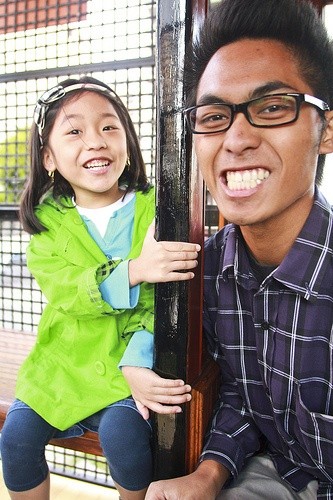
xmin=180 ymin=89 xmax=327 ymax=134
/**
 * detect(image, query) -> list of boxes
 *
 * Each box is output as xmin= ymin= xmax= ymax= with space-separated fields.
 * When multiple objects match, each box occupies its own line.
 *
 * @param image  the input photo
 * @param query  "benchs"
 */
xmin=1 ymin=328 xmax=163 ymax=463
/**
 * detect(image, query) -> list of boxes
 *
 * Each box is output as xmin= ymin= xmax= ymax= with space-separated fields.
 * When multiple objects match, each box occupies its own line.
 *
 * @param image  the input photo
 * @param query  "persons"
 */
xmin=142 ymin=0 xmax=333 ymax=500
xmin=0 ymin=75 xmax=201 ymax=500
